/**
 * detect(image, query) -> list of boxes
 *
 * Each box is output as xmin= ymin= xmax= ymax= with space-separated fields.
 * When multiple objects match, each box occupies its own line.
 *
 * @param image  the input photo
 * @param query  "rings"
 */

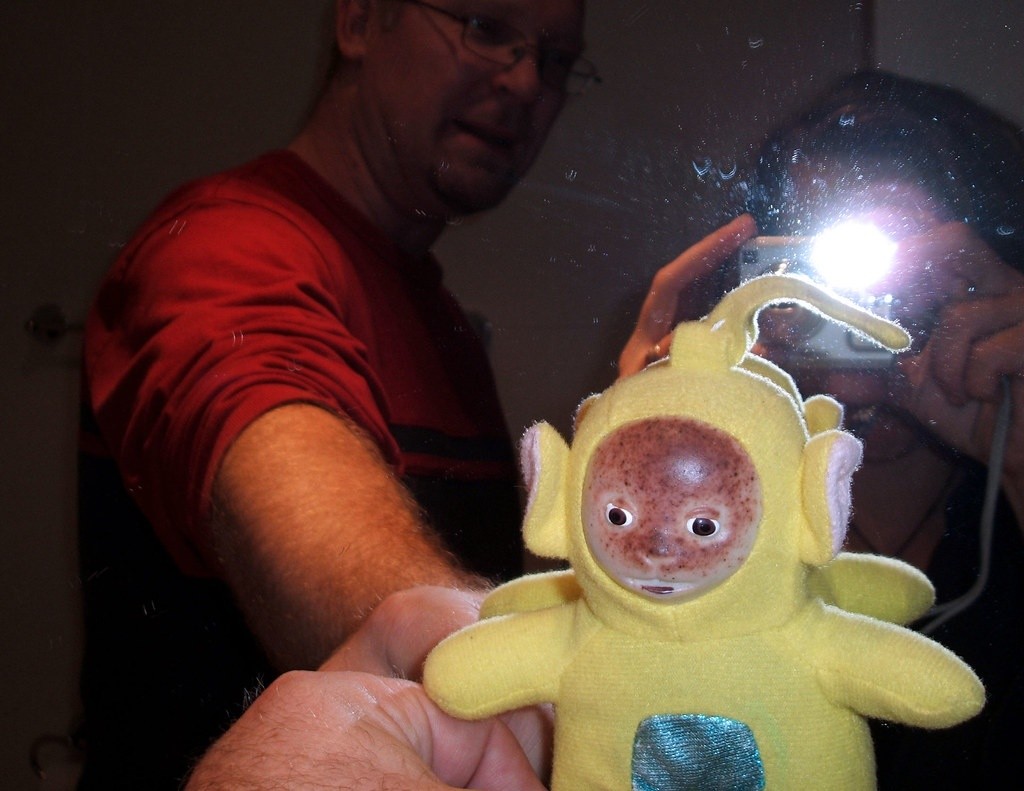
xmin=648 ymin=342 xmax=662 ymax=362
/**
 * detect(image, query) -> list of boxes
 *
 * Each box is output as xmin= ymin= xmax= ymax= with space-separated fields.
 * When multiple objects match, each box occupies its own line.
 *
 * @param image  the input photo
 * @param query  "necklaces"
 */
xmin=848 ymin=459 xmax=960 ymax=556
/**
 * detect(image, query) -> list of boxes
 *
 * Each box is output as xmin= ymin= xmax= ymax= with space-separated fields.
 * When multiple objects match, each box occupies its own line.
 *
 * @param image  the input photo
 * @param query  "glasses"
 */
xmin=409 ymin=0 xmax=603 ymax=95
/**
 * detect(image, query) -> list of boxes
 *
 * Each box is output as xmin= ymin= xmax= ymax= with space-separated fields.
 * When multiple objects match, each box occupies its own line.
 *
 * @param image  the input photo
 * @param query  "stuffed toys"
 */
xmin=477 ymin=307 xmax=937 ymax=625
xmin=424 ymin=274 xmax=986 ymax=791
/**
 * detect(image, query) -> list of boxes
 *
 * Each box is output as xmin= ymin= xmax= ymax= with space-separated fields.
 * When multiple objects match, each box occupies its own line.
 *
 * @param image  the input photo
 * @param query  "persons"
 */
xmin=86 ymin=3 xmax=1024 ymax=791
xmin=184 ymin=669 xmax=549 ymax=791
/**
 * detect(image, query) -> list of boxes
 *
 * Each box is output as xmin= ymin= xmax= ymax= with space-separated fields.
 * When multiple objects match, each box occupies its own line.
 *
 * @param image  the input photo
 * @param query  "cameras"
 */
xmin=731 ymin=233 xmax=901 ymax=368
